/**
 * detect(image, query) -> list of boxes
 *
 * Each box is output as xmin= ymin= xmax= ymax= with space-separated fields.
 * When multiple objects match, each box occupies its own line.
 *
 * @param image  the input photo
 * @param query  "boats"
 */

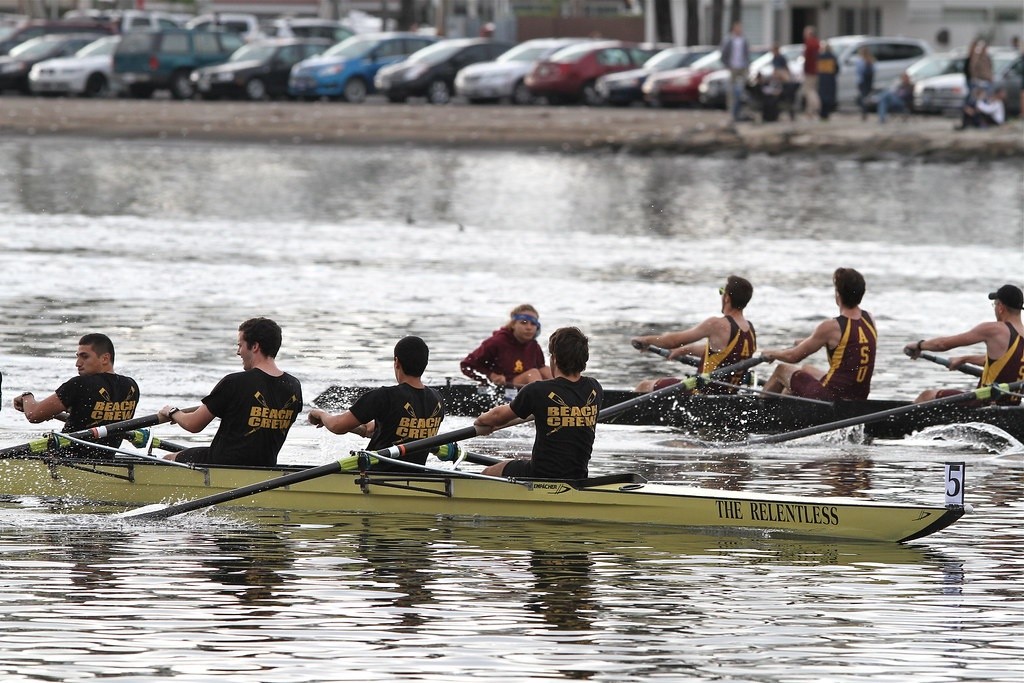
xmin=0 ymin=447 xmax=973 ymax=542
xmin=311 ymin=386 xmax=1024 ymax=447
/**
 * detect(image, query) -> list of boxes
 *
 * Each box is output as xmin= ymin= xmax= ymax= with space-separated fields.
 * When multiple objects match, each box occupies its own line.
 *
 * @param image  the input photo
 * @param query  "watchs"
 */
xmin=168 ymin=407 xmax=180 ymax=422
xmin=22 ymin=392 xmax=34 ymax=398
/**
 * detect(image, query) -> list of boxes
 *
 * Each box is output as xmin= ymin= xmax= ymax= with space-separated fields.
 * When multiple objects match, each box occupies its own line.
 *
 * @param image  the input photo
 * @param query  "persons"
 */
xmin=856 ymin=48 xmax=913 ymax=123
xmin=903 ymin=284 xmax=1024 ymax=406
xmin=761 ymin=268 xmax=877 ymax=401
xmin=461 ymin=305 xmax=553 ymax=384
xmin=721 ymin=23 xmax=839 ymax=123
xmin=308 ymin=336 xmax=445 ymax=472
xmin=635 ymin=276 xmax=757 ymax=395
xmin=473 ymin=327 xmax=603 ymax=479
xmin=958 ymin=38 xmax=1007 ymax=127
xmin=158 ymin=318 xmax=303 ymax=467
xmin=13 ymin=333 xmax=139 ymax=459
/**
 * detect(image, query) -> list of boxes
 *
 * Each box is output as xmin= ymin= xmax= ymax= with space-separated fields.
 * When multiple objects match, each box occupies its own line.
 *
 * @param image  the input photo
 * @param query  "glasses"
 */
xmin=719 ymin=288 xmax=729 ymax=296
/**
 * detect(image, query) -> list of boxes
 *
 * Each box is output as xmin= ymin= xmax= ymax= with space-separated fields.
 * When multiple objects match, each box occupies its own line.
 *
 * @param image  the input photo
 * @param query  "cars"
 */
xmin=762 ymin=35 xmax=933 ymax=105
xmin=0 ymin=14 xmax=120 ymax=85
xmin=891 ymin=44 xmax=1024 ymax=116
xmin=189 ymin=37 xmax=333 ymax=101
xmin=594 ymin=46 xmax=716 ymax=100
xmin=642 ymin=48 xmax=767 ymax=104
xmin=455 ymin=37 xmax=593 ymax=105
xmin=30 ymin=35 xmax=121 ymax=97
xmin=525 ymin=41 xmax=662 ymax=98
xmin=374 ymin=38 xmax=516 ymax=105
xmin=698 ymin=44 xmax=806 ymax=107
xmin=67 ymin=8 xmax=358 ymax=41
xmin=112 ymin=29 xmax=246 ymax=101
xmin=288 ymin=31 xmax=443 ymax=103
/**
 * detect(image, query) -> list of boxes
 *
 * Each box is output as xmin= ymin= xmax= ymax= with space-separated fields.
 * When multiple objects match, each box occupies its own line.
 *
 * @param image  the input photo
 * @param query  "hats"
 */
xmin=988 ymin=285 xmax=1024 ymax=309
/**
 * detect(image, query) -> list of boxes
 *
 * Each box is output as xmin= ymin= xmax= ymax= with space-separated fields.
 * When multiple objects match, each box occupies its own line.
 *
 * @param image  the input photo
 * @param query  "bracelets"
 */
xmin=918 ymin=339 xmax=925 ymax=350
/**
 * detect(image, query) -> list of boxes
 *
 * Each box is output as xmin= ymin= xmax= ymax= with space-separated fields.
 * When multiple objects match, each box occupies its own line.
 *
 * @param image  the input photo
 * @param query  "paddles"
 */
xmin=658 ymin=379 xmax=1024 ymax=450
xmin=595 ymin=345 xmax=796 ymax=422
xmin=903 ymin=348 xmax=983 ymax=378
xmin=0 ymin=403 xmax=203 ymax=462
xmin=116 ymin=412 xmax=535 ymax=524
xmin=632 ymin=341 xmax=768 ymax=387
xmin=310 ymin=415 xmax=502 ymax=466
xmin=20 ymin=401 xmax=186 ymax=452
